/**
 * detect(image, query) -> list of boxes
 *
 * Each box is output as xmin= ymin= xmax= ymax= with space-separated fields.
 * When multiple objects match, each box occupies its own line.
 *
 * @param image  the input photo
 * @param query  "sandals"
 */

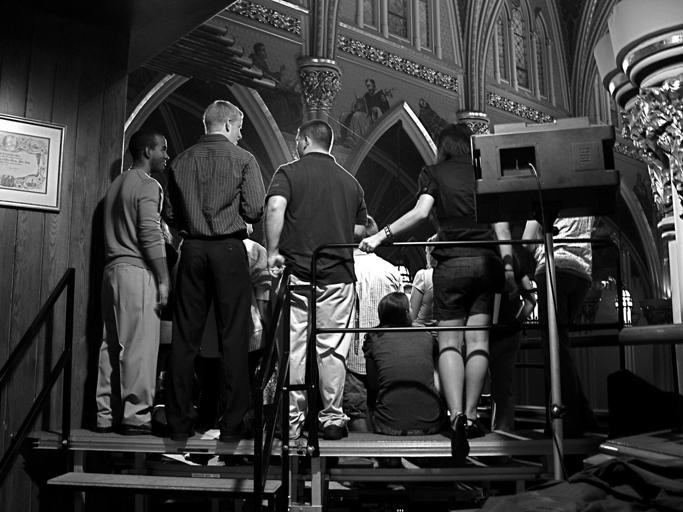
xmin=451 ymin=414 xmax=469 ymax=462
xmin=467 ymin=418 xmax=484 ymax=438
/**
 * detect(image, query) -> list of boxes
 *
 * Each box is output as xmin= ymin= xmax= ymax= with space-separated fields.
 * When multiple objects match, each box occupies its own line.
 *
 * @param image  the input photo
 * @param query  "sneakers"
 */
xmin=323 ymin=425 xmax=346 ymax=440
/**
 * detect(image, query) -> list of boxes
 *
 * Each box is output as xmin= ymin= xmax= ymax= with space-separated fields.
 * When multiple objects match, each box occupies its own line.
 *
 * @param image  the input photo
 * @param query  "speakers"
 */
xmin=470 ymin=116 xmax=620 ymax=224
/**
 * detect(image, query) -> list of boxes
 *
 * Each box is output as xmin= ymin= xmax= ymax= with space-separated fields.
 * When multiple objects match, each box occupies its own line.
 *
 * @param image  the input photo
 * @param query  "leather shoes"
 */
xmin=172 ymin=431 xmax=196 ymax=440
xmin=97 ymin=418 xmax=169 ymax=436
xmin=218 ymin=431 xmax=252 ymax=442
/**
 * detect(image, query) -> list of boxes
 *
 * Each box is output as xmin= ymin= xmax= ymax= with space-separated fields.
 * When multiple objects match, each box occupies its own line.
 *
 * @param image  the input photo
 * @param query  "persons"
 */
xmin=261 ymin=118 xmax=371 ymax=442
xmin=151 ymin=202 xmax=538 ymax=437
xmin=248 ymin=42 xmax=281 ymax=82
xmin=513 ymin=204 xmax=596 ymax=439
xmin=158 ymin=99 xmax=267 ymax=440
xmin=349 ymin=122 xmax=505 ymax=459
xmin=90 ymin=130 xmax=179 ymax=432
xmin=352 ymin=78 xmax=391 ymax=120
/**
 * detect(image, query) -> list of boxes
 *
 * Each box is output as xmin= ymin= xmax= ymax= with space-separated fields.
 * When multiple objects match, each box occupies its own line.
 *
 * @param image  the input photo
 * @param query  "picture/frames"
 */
xmin=0 ymin=113 xmax=68 ymax=213
